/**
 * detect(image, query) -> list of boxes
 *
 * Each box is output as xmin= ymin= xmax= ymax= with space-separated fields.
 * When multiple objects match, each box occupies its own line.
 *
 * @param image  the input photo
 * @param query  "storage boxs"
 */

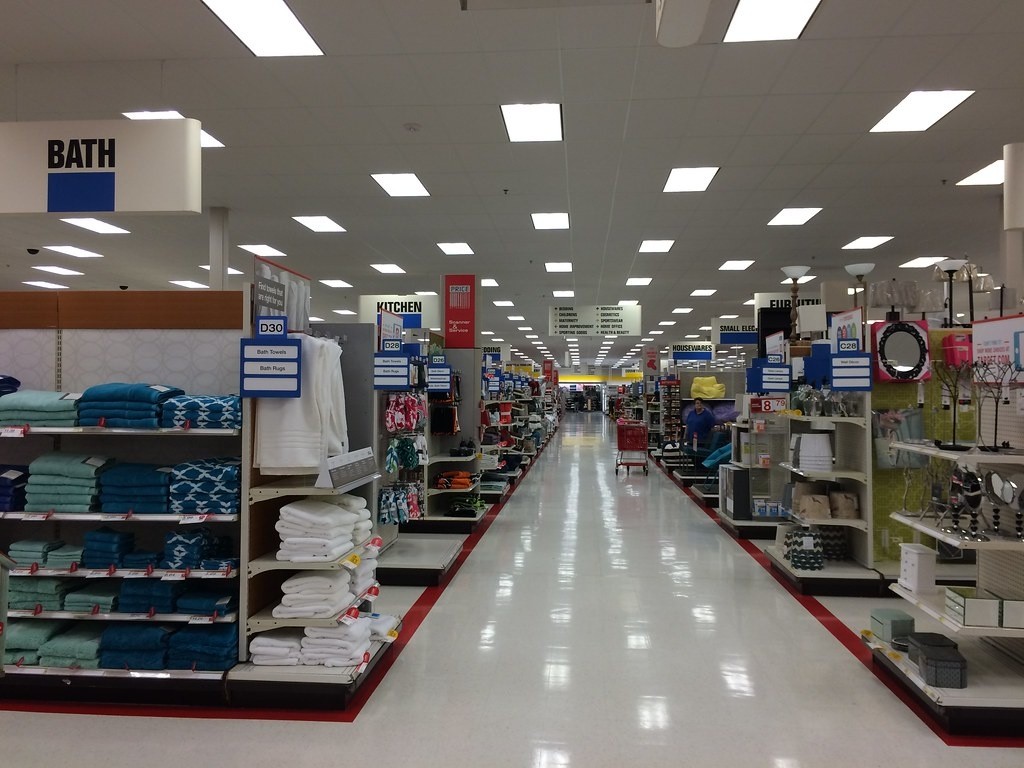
xmin=869 ymin=608 xmax=968 ymax=689
xmin=941 ymin=585 xmax=1024 ymax=630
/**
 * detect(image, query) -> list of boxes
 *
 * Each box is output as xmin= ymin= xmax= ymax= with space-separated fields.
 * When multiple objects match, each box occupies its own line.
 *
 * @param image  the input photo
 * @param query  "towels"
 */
xmin=248 ymin=493 xmax=399 ymax=667
xmin=0 ymin=376 xmax=242 ymax=672
xmin=251 ymin=334 xmax=350 ymax=475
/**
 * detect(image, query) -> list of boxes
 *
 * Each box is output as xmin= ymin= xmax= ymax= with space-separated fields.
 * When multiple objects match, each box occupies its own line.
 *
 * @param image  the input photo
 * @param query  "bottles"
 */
xmin=837 ymin=326 xmax=842 ymax=338
xmin=851 ymin=322 xmax=856 ymax=338
xmin=842 ymin=325 xmax=847 ymax=338
xmin=847 ymin=324 xmax=851 ymax=338
xmin=791 ymin=376 xmax=865 ymax=417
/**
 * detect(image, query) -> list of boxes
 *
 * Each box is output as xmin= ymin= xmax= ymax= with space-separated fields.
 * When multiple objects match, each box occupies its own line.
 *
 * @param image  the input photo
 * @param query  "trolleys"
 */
xmin=615 ymin=418 xmax=649 ymax=476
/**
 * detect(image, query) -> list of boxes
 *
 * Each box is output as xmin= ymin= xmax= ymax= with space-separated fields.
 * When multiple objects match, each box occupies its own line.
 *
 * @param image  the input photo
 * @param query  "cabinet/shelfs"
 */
xmin=298 ymin=311 xmax=564 ymax=590
xmin=0 ymin=282 xmax=405 ymax=713
xmin=857 ymin=312 xmax=1024 ymax=745
xmin=607 ymin=279 xmax=978 ymax=605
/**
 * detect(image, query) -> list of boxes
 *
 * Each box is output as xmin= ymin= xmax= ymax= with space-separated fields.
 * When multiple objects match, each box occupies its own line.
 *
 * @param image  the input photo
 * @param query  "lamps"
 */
xmin=780 ymin=260 xmax=995 ymax=342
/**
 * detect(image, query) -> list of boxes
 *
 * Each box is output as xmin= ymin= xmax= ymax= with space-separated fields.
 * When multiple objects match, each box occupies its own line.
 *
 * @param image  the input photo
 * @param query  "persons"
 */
xmin=574 ymin=395 xmax=585 ymax=413
xmin=609 ymin=397 xmax=614 ymax=415
xmin=683 ymin=397 xmax=716 ymax=442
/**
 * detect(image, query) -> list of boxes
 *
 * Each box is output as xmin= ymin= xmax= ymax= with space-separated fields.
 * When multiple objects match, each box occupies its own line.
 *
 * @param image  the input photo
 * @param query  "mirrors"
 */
xmin=875 ymin=319 xmax=930 ymax=382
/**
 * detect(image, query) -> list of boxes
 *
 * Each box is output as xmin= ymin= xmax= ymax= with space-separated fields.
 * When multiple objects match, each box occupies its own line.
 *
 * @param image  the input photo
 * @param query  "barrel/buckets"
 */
xmin=789 ymin=433 xmax=833 ymax=470
xmin=775 ymin=524 xmax=851 ymax=572
xmin=781 ymin=479 xmax=860 ymax=520
